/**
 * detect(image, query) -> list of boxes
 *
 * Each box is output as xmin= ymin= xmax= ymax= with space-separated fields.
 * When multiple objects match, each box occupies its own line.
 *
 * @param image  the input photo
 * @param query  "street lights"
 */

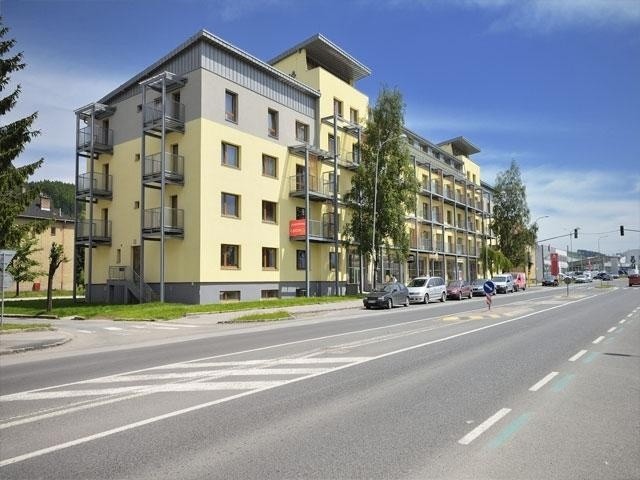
xmin=598 ymin=235 xmax=609 ymax=257
xmin=534 ymin=215 xmax=549 ymax=233
xmin=368 ymin=132 xmax=408 ymax=295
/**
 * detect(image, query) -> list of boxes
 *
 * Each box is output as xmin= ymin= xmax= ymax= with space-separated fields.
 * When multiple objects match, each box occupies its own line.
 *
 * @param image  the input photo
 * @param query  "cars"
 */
xmin=560 ymin=268 xmax=629 ymax=285
xmin=361 ymin=272 xmax=527 ymax=309
xmin=627 ymin=273 xmax=640 ymax=287
xmin=541 ymin=275 xmax=560 ymax=288
